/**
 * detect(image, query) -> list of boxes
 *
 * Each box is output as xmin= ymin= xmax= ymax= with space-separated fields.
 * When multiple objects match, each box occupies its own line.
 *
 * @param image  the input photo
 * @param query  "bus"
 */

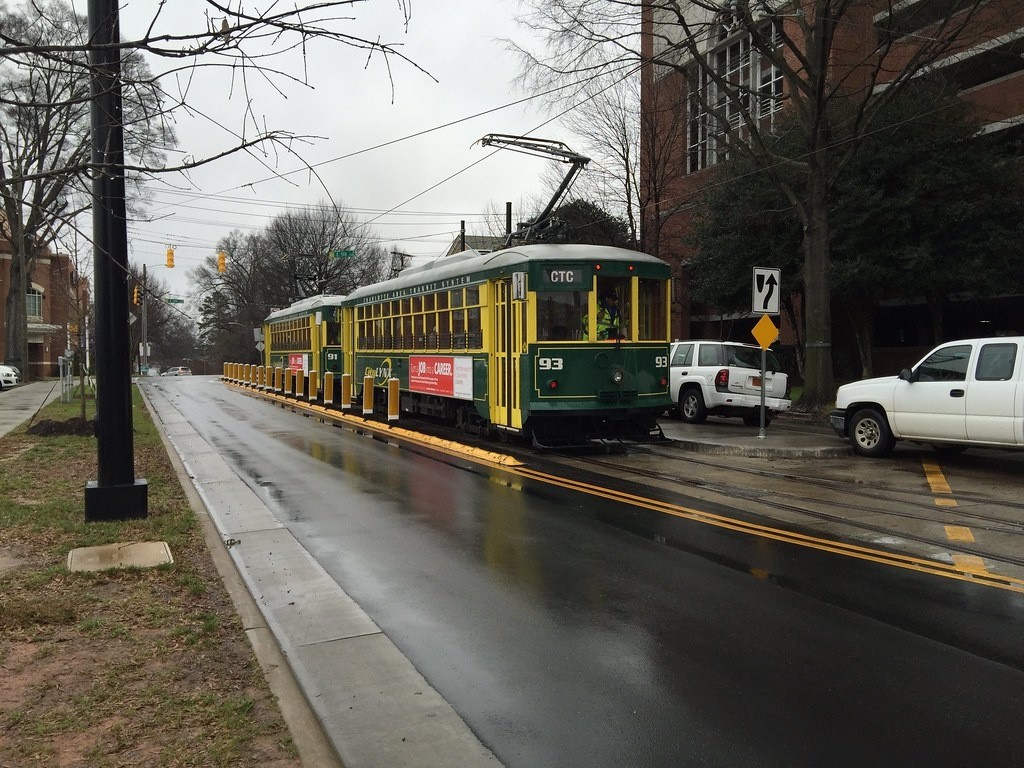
xmin=263 ymin=241 xmax=678 ymax=452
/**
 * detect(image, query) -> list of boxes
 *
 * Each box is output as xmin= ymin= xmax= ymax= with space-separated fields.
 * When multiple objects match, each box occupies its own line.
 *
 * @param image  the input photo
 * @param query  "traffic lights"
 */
xmin=134 ymin=287 xmax=142 ymax=307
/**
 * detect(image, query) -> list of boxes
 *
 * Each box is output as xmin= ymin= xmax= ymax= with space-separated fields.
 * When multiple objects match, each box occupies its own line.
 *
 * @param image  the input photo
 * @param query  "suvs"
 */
xmin=667 ymin=338 xmax=792 ymax=426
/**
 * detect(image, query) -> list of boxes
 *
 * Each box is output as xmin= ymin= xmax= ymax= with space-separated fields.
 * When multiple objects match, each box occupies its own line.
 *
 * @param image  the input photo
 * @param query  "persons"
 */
xmin=582 ymin=291 xmax=629 ymax=341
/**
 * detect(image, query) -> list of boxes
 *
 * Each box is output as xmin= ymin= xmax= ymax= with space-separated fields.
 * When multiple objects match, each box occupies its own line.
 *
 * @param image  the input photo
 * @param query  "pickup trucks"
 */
xmin=831 ymin=336 xmax=1024 ymax=453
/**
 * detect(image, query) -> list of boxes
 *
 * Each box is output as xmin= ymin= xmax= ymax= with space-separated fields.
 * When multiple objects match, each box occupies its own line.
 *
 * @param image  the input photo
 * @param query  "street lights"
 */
xmin=142 ymin=264 xmax=175 ymax=375
xmin=226 ymin=320 xmax=261 ymax=365
xmin=194 ymin=347 xmax=207 ymax=375
xmin=19 ymin=233 xmax=37 ymax=383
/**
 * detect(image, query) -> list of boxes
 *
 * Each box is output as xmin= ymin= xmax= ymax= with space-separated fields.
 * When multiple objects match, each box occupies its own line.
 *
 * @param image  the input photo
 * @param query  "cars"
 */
xmin=0 ymin=359 xmax=22 ymax=391
xmin=161 ymin=366 xmax=192 ymax=376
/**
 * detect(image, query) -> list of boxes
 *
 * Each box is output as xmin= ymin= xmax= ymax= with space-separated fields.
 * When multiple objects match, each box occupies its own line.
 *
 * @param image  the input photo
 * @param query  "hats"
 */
xmin=605 ymin=289 xmax=621 ymax=300
